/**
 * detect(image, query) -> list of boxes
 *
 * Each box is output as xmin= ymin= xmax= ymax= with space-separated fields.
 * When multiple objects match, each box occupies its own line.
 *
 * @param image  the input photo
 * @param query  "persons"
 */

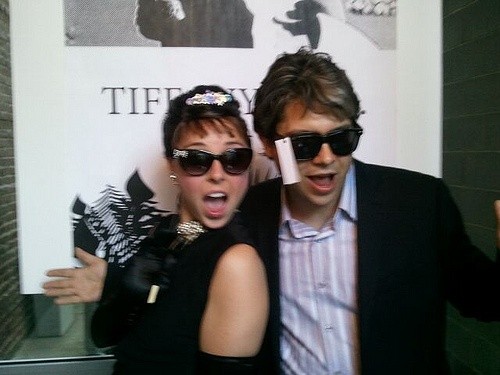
xmin=89 ymin=85 xmax=275 ymax=375
xmin=43 ymin=46 xmax=500 ymax=375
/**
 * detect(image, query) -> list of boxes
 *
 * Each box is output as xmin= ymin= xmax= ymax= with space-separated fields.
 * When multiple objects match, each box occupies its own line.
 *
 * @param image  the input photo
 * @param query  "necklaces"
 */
xmin=175 ymin=220 xmax=205 ymax=236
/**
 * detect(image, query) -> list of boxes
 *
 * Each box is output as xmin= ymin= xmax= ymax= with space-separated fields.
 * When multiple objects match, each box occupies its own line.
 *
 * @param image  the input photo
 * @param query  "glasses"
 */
xmin=266 ymin=120 xmax=364 ymax=161
xmin=168 ymin=147 xmax=253 ymax=175
